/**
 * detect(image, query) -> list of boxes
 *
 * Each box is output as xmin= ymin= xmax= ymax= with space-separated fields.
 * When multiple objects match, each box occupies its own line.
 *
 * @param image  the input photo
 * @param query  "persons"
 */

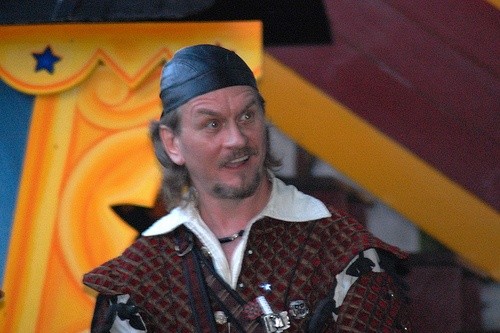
xmin=103 ymin=44 xmax=408 ymax=333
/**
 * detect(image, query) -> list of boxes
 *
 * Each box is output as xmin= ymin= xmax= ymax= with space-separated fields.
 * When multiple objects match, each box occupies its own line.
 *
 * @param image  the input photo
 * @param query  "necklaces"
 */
xmin=213 ymin=228 xmax=245 ymax=244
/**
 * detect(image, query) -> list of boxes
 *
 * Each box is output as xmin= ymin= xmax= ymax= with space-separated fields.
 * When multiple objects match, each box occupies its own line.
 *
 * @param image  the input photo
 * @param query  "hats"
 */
xmin=159 ymin=44 xmax=258 ymax=112
